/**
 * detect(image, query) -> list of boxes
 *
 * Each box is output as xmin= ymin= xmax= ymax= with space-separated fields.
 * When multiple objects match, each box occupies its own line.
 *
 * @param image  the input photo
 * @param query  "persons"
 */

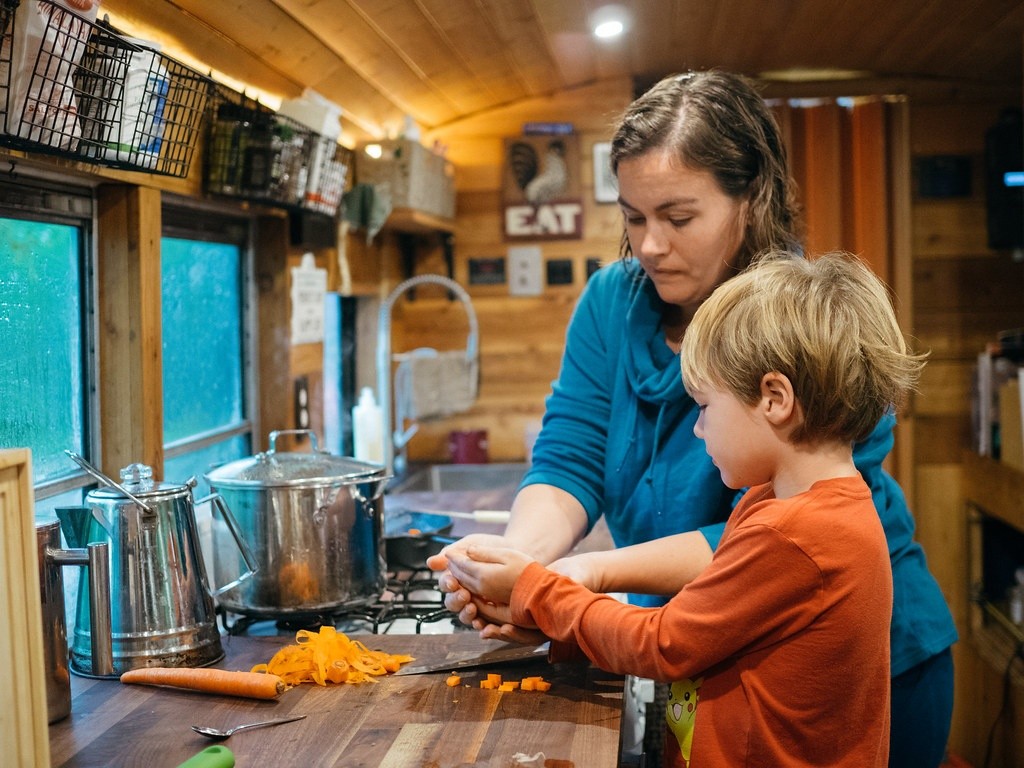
xmin=427 ymin=66 xmax=958 ymax=768
xmin=442 ymin=240 xmax=937 ymax=767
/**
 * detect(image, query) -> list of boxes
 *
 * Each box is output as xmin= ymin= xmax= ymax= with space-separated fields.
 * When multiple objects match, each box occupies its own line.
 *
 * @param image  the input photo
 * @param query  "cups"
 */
xmin=35 ymin=515 xmax=114 ymax=726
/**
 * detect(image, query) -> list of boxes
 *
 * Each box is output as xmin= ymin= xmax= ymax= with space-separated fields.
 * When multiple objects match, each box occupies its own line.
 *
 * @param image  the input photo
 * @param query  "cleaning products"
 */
xmin=352 ymin=385 xmax=385 ymax=466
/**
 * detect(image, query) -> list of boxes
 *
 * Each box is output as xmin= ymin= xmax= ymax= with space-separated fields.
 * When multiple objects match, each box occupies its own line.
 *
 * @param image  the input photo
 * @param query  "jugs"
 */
xmin=55 ymin=448 xmax=262 ymax=682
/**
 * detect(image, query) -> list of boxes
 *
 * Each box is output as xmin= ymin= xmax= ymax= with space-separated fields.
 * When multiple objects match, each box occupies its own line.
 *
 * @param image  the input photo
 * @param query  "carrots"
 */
xmin=120 ymin=667 xmax=285 ymax=700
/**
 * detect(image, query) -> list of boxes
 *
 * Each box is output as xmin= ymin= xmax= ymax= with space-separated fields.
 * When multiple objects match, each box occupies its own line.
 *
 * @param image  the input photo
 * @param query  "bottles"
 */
xmin=78 ymin=33 xmax=173 ymax=171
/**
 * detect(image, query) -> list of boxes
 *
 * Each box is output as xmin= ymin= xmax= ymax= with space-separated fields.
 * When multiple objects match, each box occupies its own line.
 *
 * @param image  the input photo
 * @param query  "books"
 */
xmin=972 ymin=334 xmax=1024 ymax=472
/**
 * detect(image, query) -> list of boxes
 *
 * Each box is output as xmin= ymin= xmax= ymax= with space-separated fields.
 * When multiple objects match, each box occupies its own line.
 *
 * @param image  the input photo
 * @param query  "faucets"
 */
xmin=371 ymin=277 xmax=484 ymax=396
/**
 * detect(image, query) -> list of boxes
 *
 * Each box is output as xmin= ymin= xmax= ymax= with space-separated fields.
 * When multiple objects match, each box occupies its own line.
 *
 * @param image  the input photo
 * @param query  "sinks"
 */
xmin=382 ymin=464 xmax=533 ymax=516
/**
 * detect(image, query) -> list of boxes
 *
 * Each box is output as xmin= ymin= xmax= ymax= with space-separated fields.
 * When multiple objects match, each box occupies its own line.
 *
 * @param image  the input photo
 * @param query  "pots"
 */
xmin=201 ymin=429 xmax=395 ymax=635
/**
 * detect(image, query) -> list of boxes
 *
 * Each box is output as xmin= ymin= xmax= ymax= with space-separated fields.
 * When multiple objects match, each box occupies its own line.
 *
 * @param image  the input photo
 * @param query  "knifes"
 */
xmin=392 ymin=639 xmax=551 ymax=677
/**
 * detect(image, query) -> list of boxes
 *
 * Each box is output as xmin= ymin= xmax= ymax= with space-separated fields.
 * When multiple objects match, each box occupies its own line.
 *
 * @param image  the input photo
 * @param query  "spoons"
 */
xmin=190 ymin=715 xmax=307 ymax=742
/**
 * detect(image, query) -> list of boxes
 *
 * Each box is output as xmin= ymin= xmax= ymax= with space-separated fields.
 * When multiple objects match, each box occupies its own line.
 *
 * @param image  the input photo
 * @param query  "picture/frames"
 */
xmin=0 ymin=448 xmax=50 ymax=768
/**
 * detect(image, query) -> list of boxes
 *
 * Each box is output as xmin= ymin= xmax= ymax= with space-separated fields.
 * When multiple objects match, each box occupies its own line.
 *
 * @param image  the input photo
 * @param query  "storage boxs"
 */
xmin=203 ymin=70 xmax=350 ymax=227
xmin=354 ymin=137 xmax=460 ymax=236
xmin=0 ymin=0 xmax=218 ymax=180
